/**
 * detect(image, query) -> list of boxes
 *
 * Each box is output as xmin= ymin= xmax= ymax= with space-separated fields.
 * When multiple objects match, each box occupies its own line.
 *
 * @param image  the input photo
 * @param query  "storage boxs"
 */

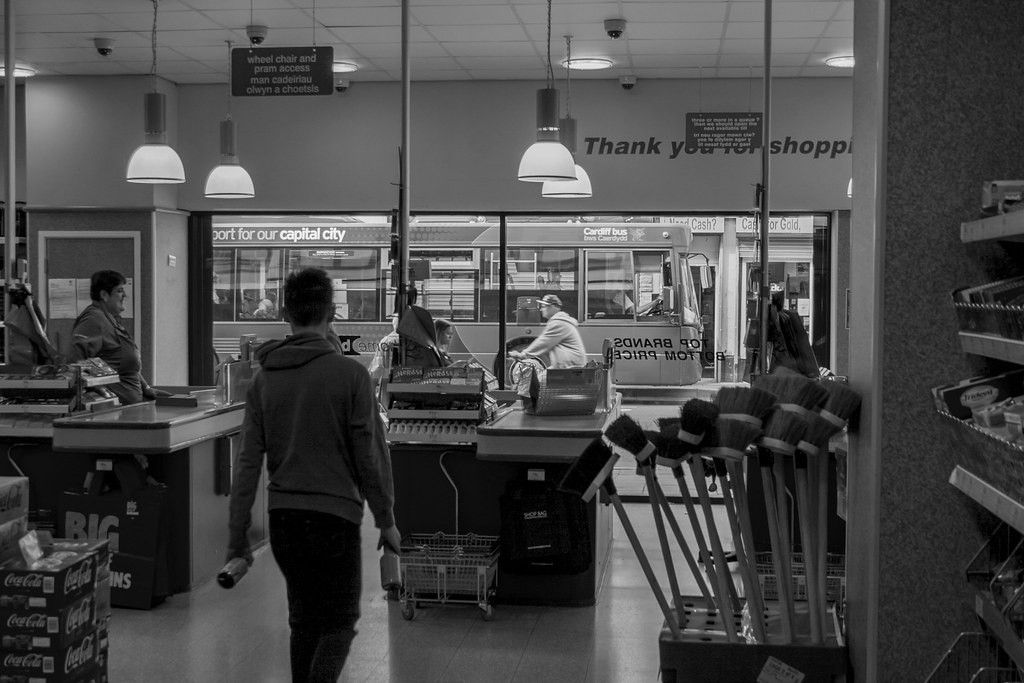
xmin=547 ymin=361 xmax=610 ymax=410
xmin=0 ymin=476 xmax=110 ymax=683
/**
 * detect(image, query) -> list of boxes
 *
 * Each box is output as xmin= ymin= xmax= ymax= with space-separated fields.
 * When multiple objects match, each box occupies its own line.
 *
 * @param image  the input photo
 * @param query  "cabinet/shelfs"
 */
xmin=384 ymin=368 xmax=486 ymax=446
xmin=945 ymin=207 xmax=1024 ymax=683
xmin=0 ymin=365 xmax=77 ymax=437
xmin=70 ymin=360 xmax=123 ymax=413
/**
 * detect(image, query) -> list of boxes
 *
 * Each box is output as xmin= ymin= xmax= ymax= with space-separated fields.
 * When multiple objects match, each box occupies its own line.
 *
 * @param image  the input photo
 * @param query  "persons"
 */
xmin=253 ymin=299 xmax=274 ymax=319
xmin=613 ymin=273 xmax=661 ymax=316
xmin=368 ymin=318 xmax=401 ymax=413
xmin=68 ymin=270 xmax=173 ymax=405
xmin=433 ymin=319 xmax=453 ymax=364
xmin=225 ymin=268 xmax=401 ymax=683
xmin=507 ymin=294 xmax=588 ymax=383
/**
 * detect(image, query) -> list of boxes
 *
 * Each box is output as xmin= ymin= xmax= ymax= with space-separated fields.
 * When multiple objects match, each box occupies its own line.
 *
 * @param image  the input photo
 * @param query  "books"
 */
xmin=447 ymin=355 xmax=476 ymax=368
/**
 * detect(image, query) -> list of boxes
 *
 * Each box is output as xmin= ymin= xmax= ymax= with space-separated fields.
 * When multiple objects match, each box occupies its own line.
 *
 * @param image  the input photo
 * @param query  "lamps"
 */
xmin=333 ymin=60 xmax=358 ymax=73
xmin=126 ymin=0 xmax=185 ymax=185
xmin=542 ymin=35 xmax=593 ymax=198
xmin=562 ymin=57 xmax=614 ymax=69
xmin=517 ymin=0 xmax=578 ymax=182
xmin=0 ymin=66 xmax=36 ymax=77
xmin=205 ymin=39 xmax=256 ymax=200
xmin=825 ymin=55 xmax=854 ymax=68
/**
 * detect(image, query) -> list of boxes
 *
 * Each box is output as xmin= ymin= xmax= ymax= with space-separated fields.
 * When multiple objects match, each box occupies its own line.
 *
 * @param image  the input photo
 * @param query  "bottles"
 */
xmin=380 ymin=543 xmax=403 ymax=590
xmin=217 ymin=556 xmax=250 ymax=589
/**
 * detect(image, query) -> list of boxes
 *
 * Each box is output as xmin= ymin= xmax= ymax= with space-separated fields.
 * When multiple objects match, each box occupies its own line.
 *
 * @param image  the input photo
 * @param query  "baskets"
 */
xmin=395 ymin=531 xmax=502 ymax=596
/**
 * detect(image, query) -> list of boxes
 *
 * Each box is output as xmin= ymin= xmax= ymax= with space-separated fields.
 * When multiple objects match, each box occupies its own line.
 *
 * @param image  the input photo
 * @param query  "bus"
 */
xmin=42 ymin=213 xmax=715 ymax=394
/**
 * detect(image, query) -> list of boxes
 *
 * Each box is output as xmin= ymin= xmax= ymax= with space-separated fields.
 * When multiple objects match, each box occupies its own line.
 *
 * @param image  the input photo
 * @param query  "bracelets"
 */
xmin=144 ymin=385 xmax=151 ymax=391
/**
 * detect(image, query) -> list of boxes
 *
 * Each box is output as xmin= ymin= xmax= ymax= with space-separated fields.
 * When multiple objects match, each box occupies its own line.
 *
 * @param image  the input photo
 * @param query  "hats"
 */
xmin=535 ymin=294 xmax=563 ymax=308
